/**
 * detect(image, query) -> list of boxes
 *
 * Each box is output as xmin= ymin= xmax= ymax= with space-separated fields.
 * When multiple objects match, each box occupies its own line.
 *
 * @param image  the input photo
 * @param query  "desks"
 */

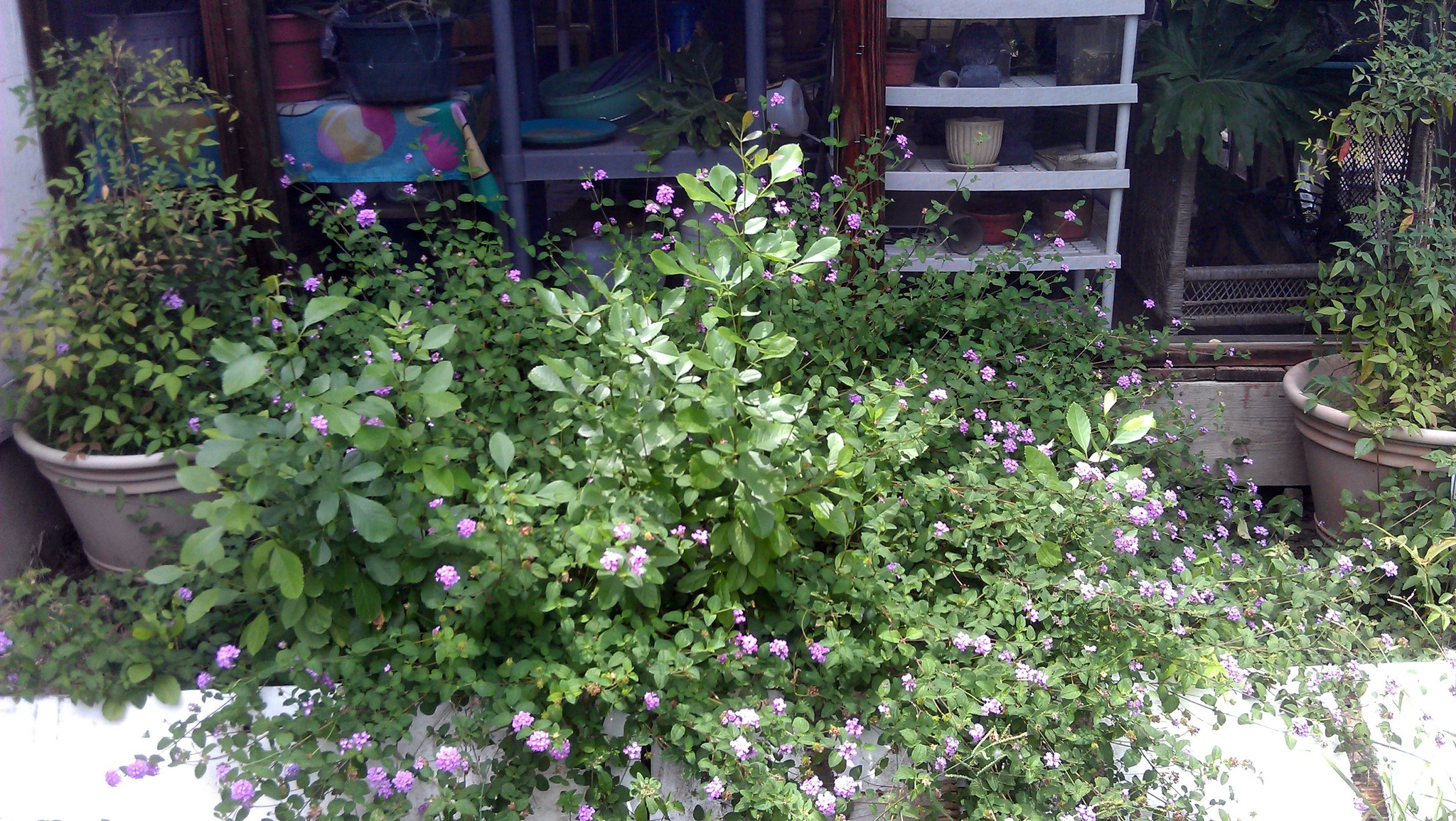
xmin=73 ymin=73 xmax=498 ymax=251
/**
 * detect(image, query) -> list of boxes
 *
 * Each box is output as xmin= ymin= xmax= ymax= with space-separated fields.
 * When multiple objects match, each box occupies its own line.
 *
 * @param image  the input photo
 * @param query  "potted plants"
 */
xmin=1280 ymin=0 xmax=1456 ymax=556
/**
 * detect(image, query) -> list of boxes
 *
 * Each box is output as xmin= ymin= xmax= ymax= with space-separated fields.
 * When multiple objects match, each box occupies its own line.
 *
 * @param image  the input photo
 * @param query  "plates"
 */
xmin=521 ymin=118 xmax=617 ymax=143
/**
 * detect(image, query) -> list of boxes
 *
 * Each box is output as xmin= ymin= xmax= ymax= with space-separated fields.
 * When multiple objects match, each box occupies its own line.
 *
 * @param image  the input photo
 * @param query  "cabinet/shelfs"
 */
xmin=486 ymin=0 xmax=774 ymax=303
xmin=880 ymin=0 xmax=1147 ymax=339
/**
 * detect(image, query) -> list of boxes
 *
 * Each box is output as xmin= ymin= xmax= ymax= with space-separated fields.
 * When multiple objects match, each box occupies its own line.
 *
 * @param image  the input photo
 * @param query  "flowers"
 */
xmin=0 ymin=39 xmax=346 ymax=463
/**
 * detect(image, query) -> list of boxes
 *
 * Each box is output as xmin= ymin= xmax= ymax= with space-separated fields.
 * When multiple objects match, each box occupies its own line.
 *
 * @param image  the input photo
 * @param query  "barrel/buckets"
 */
xmin=679 ymin=198 xmax=751 ymax=292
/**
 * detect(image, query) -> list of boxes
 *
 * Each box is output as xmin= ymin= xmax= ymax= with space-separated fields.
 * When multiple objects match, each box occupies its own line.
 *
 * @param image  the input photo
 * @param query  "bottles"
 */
xmin=766 ymin=78 xmax=805 ymax=137
xmin=663 ymin=5 xmax=705 ymax=84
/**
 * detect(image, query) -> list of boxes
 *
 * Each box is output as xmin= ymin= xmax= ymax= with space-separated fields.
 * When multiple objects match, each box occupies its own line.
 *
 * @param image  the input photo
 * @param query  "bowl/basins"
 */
xmin=960 ymin=199 xmax=1029 ymax=243
xmin=945 ymin=117 xmax=1004 ymax=165
xmin=884 ymin=48 xmax=921 ymax=86
xmin=539 ymin=50 xmax=659 ymax=118
xmin=1042 ymin=191 xmax=1094 ymax=241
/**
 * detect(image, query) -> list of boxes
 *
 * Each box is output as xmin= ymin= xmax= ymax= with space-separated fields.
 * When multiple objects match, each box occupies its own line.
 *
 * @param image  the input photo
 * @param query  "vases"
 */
xmin=12 ymin=367 xmax=233 ymax=588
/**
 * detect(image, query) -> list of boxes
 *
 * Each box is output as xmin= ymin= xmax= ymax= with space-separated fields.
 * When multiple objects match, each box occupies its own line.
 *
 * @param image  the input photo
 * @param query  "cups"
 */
xmin=937 ymin=211 xmax=984 ymax=255
